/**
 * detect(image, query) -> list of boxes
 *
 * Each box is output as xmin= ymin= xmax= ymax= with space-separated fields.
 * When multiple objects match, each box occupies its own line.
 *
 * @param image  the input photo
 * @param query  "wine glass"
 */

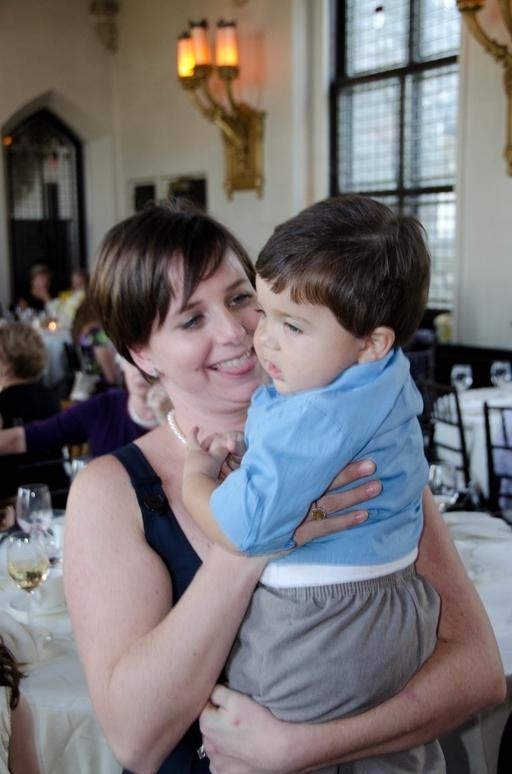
xmin=5 ymin=531 xmax=53 ymax=644
xmin=490 ymin=362 xmax=510 ymax=398
xmin=451 ymin=364 xmax=472 ymax=403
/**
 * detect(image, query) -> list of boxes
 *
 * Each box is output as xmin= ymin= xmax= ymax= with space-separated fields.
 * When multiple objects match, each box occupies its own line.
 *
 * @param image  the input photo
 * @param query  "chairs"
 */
xmin=416 ymin=378 xmax=470 ymax=487
xmin=483 ymin=401 xmax=511 ymax=515
xmin=404 ymin=350 xmax=435 ymax=384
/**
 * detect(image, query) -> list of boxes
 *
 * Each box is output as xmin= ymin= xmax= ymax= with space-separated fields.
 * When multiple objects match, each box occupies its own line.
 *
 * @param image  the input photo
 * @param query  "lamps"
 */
xmin=175 ymin=19 xmax=268 ymax=202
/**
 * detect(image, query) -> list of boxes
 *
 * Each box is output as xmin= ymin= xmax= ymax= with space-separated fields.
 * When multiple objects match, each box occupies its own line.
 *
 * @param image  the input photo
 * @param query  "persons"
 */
xmin=180 ymin=190 xmax=450 ymax=774
xmin=62 ymin=196 xmax=509 ymax=774
xmin=1 ymin=634 xmax=43 ymax=773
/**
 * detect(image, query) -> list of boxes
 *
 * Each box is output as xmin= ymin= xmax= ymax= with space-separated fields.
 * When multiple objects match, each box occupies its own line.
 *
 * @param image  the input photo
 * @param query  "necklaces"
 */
xmin=166 ymin=409 xmax=191 ymax=445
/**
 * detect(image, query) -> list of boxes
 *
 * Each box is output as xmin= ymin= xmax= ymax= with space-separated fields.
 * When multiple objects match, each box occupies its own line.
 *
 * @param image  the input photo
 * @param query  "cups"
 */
xmin=17 ymin=484 xmax=53 ymax=535
xmin=430 ymin=463 xmax=460 ymax=513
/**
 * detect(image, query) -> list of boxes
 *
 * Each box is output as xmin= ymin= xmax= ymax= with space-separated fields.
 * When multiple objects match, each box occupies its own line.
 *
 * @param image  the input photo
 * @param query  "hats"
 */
xmin=31 ymin=262 xmax=47 ymax=275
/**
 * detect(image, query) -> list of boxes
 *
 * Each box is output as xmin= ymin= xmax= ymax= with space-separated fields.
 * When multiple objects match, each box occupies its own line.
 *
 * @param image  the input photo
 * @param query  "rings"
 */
xmin=309 ymin=501 xmax=326 ymax=522
